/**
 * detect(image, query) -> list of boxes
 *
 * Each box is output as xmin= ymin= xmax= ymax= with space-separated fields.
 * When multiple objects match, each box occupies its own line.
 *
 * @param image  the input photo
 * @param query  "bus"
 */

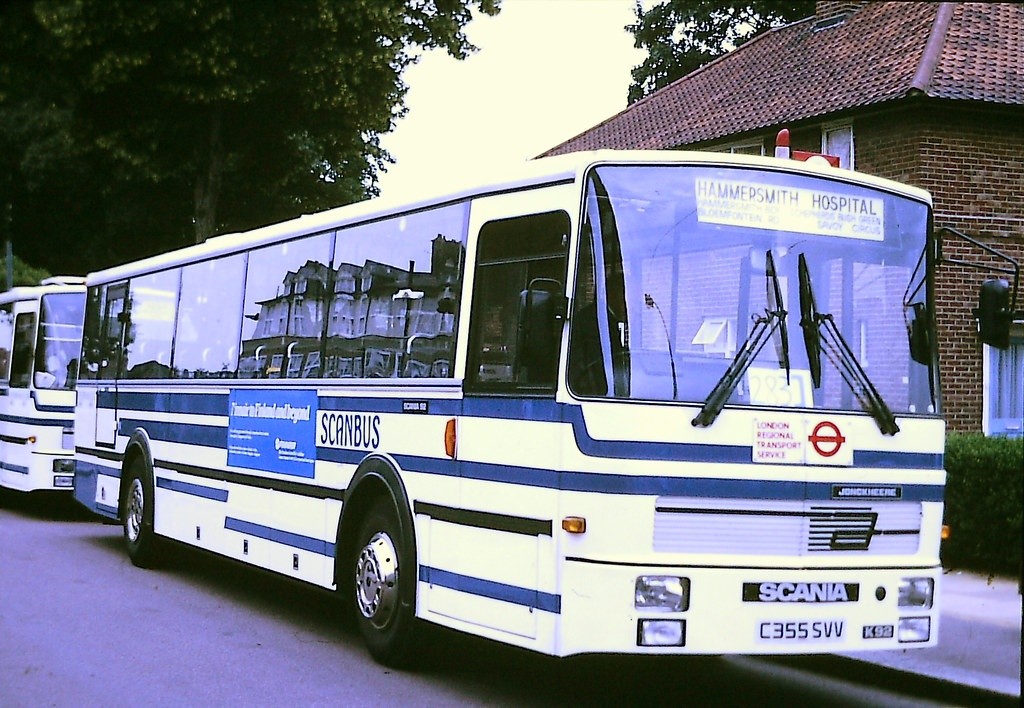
xmin=0 ymin=274 xmax=84 ymax=503
xmin=73 ymin=145 xmax=1022 ymax=669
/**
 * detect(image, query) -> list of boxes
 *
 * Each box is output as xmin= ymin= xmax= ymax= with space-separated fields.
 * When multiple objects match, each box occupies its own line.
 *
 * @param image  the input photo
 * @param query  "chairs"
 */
xmin=239 ymin=332 xmax=452 ymax=378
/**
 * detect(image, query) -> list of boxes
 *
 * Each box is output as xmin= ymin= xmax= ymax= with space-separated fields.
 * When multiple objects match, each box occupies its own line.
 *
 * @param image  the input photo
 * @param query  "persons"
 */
xmin=572 ymin=270 xmax=637 ymax=397
